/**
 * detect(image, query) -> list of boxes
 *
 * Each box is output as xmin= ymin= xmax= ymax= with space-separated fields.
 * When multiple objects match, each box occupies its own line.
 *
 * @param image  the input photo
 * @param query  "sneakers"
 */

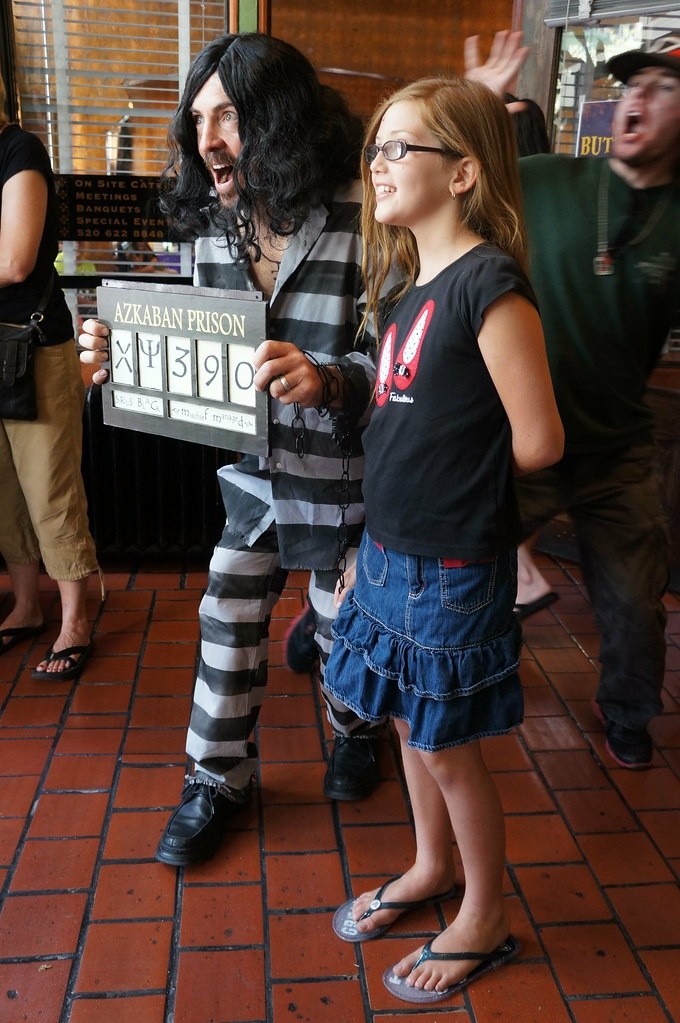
xmin=284 ymin=606 xmax=319 ymax=675
xmin=590 ymin=698 xmax=653 ymax=768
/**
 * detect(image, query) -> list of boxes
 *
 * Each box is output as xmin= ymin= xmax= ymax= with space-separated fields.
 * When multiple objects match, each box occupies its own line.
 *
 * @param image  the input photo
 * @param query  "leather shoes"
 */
xmin=156 ymin=781 xmax=252 ymax=865
xmin=322 ymin=735 xmax=383 ymax=800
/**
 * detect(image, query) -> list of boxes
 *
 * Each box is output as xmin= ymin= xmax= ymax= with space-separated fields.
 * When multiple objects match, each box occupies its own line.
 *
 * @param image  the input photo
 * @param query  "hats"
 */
xmin=609 ymin=32 xmax=680 ymax=85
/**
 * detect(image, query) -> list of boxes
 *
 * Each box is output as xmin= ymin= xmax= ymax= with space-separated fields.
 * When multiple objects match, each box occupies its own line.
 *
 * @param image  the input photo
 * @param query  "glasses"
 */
xmin=364 ymin=141 xmax=462 ymax=164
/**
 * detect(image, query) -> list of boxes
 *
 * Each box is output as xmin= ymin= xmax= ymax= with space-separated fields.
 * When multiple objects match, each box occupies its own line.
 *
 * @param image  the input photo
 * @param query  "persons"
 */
xmin=322 ymin=76 xmax=567 ymax=1004
xmin=0 ymin=77 xmax=105 ymax=684
xmin=77 ymin=31 xmax=390 ymax=866
xmin=460 ymin=28 xmax=679 ymax=773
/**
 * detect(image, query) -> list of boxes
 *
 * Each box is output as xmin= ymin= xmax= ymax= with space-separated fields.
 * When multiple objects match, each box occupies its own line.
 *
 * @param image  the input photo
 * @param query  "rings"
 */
xmin=280 ymin=375 xmax=291 ymax=391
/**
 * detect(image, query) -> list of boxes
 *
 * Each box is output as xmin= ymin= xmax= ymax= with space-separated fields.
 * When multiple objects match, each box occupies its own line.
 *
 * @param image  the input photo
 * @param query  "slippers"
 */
xmin=381 ymin=931 xmax=521 ymax=1004
xmin=0 ymin=620 xmax=46 ymax=654
xmin=512 ymin=591 xmax=554 ymax=622
xmin=330 ymin=876 xmax=461 ymax=943
xmin=31 ymin=639 xmax=94 ymax=680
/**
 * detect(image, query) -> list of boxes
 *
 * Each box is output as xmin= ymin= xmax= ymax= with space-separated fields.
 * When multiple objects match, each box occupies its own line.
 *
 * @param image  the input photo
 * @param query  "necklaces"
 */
xmin=592 ymin=154 xmax=672 ymax=275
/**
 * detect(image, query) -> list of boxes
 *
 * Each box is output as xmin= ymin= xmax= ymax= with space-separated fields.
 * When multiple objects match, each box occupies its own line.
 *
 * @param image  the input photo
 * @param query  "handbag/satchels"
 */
xmin=0 ymin=320 xmax=45 ymax=421
